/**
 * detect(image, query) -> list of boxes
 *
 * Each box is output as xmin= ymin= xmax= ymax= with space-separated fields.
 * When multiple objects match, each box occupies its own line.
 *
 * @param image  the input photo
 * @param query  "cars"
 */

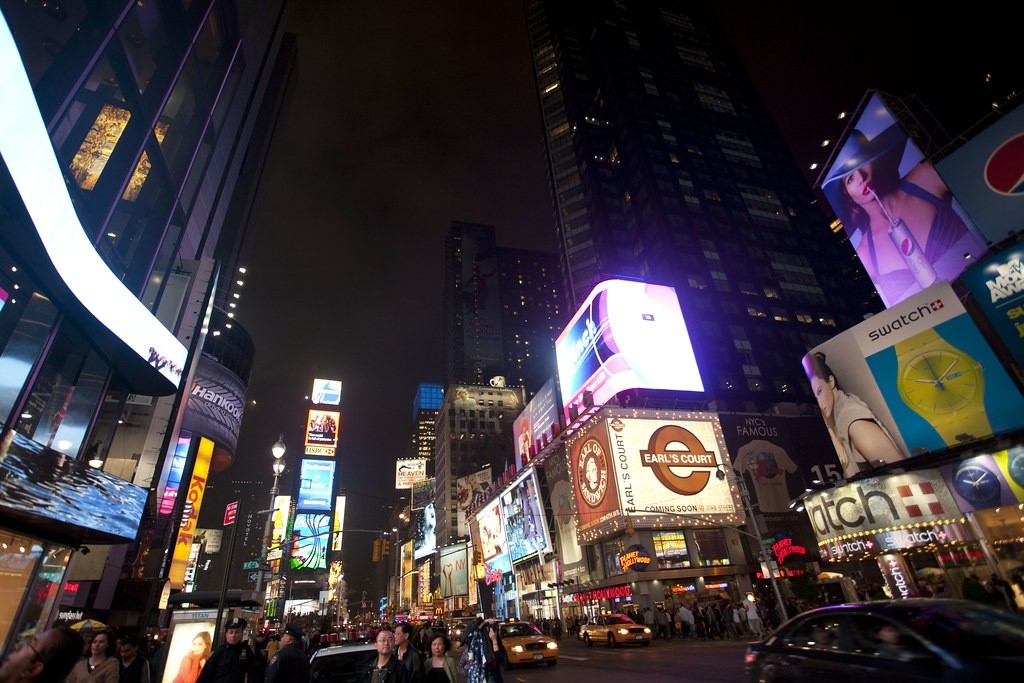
xmin=578 ymin=613 xmax=653 ymax=649
xmin=497 ymin=621 xmax=559 ymax=669
xmin=309 ymin=640 xmax=380 ymax=683
xmin=446 ymin=616 xmax=477 ymax=639
xmin=744 ymin=597 xmax=1023 ymax=683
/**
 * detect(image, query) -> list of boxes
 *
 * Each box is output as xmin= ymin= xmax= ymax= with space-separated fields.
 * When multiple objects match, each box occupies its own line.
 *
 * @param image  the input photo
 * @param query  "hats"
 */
xmin=280 ymin=623 xmax=306 ymax=639
xmin=224 ymin=618 xmax=247 ymax=631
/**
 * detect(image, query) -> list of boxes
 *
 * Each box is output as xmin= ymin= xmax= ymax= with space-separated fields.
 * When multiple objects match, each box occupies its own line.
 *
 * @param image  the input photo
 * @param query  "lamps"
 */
xmin=79 ymin=546 xmax=91 ymax=556
xmin=805 ymin=488 xmax=820 ymax=493
xmin=210 ymin=264 xmax=247 ymax=330
xmin=955 ymin=432 xmax=974 ymax=442
xmin=548 ymin=579 xmax=577 ymax=589
xmin=813 ymin=480 xmax=835 ymax=484
xmin=22 ymin=406 xmax=32 ymax=421
xmin=911 ymin=447 xmax=927 ymax=454
xmin=789 ymin=496 xmax=811 ymax=508
xmin=809 ymin=162 xmax=826 ymax=171
xmin=869 ymin=459 xmax=887 ymax=468
xmin=797 ymin=503 xmax=807 ymax=513
xmin=20 ymin=542 xmax=27 ymax=553
xmin=88 ymin=441 xmax=109 ymax=469
xmin=822 ymin=138 xmax=839 ymax=148
xmin=838 ymin=111 xmax=853 ymax=120
xmin=3 ymin=538 xmax=15 ymax=548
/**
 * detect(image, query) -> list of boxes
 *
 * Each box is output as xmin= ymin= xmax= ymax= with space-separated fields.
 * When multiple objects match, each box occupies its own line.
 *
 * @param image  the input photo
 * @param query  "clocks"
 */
xmin=900 ymin=350 xmax=977 ymax=413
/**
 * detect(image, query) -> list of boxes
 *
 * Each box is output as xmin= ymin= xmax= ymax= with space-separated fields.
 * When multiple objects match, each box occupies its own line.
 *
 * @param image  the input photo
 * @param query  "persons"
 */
xmin=617 ymin=593 xmax=802 ymax=639
xmin=856 ymin=582 xmax=890 ymax=600
xmin=803 ymin=351 xmax=906 ymax=479
xmin=809 ymin=622 xmax=840 ymax=651
xmin=962 ymin=573 xmax=1019 ymax=614
xmin=918 ymin=573 xmax=937 ymax=598
xmin=870 ymin=619 xmax=915 ymax=660
xmin=458 ymin=617 xmax=505 ymax=683
xmin=821 ymin=129 xmax=983 ymax=308
xmin=531 ymin=614 xmax=588 ymax=638
xmin=357 ymin=619 xmax=460 ymax=683
xmin=0 ymin=611 xmax=338 ymax=683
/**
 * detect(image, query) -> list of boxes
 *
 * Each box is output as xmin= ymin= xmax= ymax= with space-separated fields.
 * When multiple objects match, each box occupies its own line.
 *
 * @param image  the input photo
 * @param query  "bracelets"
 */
xmin=492 ymin=644 xmax=498 ymax=645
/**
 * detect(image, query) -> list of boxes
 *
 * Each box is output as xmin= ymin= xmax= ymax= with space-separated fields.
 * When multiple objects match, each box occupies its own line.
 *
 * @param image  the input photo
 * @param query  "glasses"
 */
xmin=376 ymin=638 xmax=395 ymax=643
xmin=18 ymin=638 xmax=44 ymax=663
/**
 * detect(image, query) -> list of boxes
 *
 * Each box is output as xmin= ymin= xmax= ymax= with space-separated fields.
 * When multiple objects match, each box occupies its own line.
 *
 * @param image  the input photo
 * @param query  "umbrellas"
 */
xmin=70 ymin=618 xmax=106 ymax=632
xmin=17 ymin=627 xmax=36 ymax=641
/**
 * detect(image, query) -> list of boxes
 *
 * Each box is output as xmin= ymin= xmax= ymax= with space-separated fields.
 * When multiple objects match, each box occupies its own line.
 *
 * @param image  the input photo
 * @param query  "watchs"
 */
xmin=952 ymin=459 xmax=1001 ymax=510
xmin=1008 ymin=445 xmax=1024 ymax=489
xmin=894 ymin=327 xmax=993 ymax=448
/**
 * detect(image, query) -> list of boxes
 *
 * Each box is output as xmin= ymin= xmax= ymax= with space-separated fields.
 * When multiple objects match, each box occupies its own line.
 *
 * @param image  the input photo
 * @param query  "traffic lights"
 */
xmin=382 ymin=539 xmax=390 ymax=556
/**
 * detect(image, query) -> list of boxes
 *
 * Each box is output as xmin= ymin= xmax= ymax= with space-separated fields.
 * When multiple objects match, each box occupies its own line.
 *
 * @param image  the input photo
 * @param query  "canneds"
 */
xmin=887 ymin=217 xmax=936 ymax=290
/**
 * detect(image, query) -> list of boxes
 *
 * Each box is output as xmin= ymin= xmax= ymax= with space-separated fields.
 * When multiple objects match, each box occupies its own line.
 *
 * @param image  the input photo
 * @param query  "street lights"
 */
xmin=716 ymin=450 xmax=789 ymax=621
xmin=256 ymin=433 xmax=288 ymax=593
